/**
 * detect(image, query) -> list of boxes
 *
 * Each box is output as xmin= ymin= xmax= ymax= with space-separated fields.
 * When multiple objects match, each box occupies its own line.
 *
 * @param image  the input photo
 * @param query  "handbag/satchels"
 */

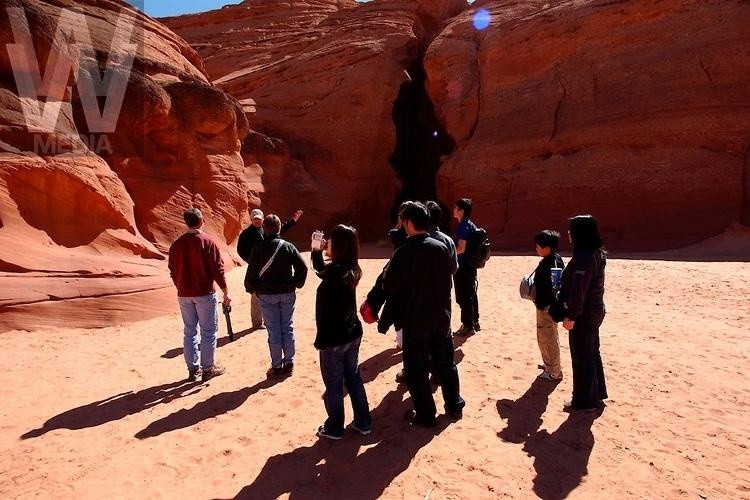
xmin=548 ymin=301 xmax=566 ymax=323
xmin=520 ymin=274 xmax=536 ymax=300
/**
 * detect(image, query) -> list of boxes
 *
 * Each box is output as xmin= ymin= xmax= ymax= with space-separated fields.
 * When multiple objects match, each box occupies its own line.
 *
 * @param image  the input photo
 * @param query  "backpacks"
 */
xmin=470 ymin=228 xmax=490 ymax=268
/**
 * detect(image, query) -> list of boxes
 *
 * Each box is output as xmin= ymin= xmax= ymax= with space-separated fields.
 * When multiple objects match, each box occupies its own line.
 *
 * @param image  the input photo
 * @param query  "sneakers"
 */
xmin=283 ymin=363 xmax=293 ymax=373
xmin=405 ymin=409 xmax=417 ymax=423
xmin=538 ymin=363 xmax=545 ymax=369
xmin=537 ymin=371 xmax=563 ymax=382
xmin=202 ymin=366 xmax=225 ymax=380
xmin=396 ymin=368 xmax=406 ymax=379
xmin=564 ymin=402 xmax=596 ymax=411
xmin=253 ymin=324 xmax=266 ymax=329
xmin=352 ymin=420 xmax=371 ymax=435
xmin=318 ymin=425 xmax=343 ymax=439
xmin=267 ymin=368 xmax=283 ymax=379
xmin=189 ymin=367 xmax=203 ymax=381
xmin=453 ymin=321 xmax=480 ymax=336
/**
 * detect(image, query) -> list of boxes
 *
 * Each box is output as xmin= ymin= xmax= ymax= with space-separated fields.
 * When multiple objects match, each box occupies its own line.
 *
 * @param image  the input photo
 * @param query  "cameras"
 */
xmin=311 ymin=232 xmax=325 ymax=242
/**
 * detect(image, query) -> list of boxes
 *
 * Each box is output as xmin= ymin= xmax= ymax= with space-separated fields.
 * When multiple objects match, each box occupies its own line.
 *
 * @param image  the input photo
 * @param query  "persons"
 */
xmin=167 ymin=207 xmax=231 ymax=383
xmin=310 ymin=224 xmax=374 ymax=441
xmin=531 ymin=229 xmax=564 ymax=383
xmin=550 ymin=214 xmax=609 ymax=413
xmin=237 ymin=208 xmax=306 ymax=330
xmin=359 ymin=197 xmax=482 ymax=428
xmin=244 ymin=213 xmax=308 ymax=383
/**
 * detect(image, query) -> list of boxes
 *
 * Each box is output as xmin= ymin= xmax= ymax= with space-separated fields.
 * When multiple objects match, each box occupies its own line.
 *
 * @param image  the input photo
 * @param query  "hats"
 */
xmin=251 ymin=209 xmax=264 ymax=220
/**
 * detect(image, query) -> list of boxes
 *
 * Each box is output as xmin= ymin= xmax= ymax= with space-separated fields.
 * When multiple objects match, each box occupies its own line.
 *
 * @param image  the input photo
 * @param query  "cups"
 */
xmin=550 ymin=268 xmax=562 ymax=287
